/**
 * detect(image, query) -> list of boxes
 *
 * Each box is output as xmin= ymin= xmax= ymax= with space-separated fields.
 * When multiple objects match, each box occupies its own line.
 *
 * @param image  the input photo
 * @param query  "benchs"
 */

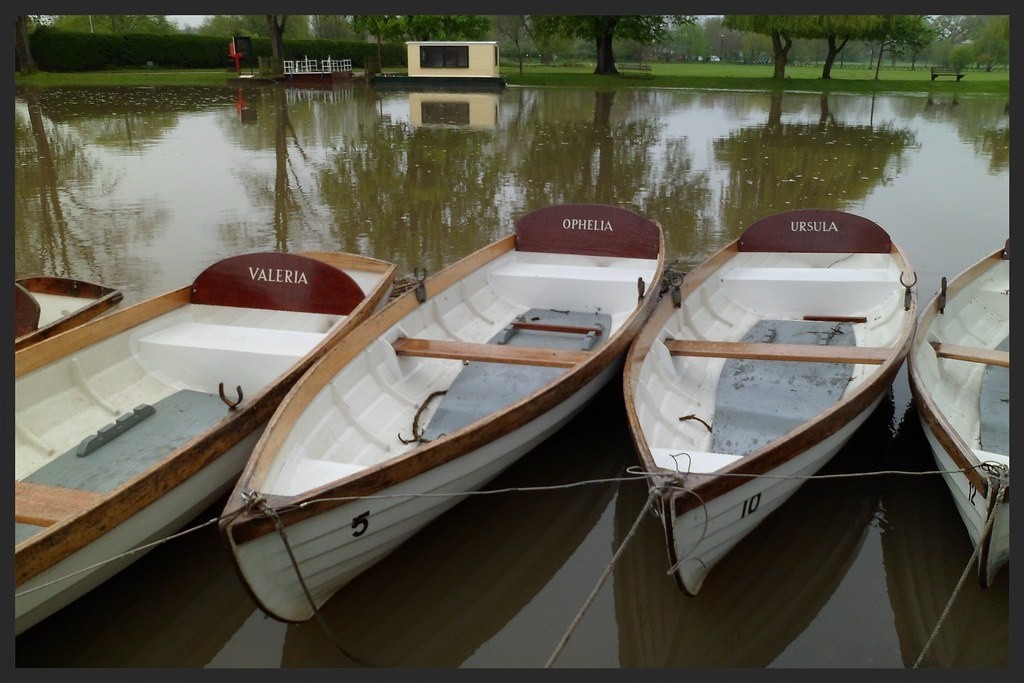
xmin=393 ymin=338 xmax=593 ymax=368
xmin=618 ymin=64 xmax=652 ymax=80
xmin=931 ymin=67 xmax=966 ymax=82
xmin=15 ymin=480 xmax=107 ymax=526
xmin=664 ymin=336 xmax=894 ymax=363
xmin=930 ymin=341 xmax=1009 ymax=368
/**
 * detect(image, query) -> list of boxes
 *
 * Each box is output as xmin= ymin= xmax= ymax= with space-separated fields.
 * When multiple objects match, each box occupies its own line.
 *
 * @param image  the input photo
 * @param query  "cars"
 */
xmin=711 ymin=56 xmax=720 ymax=62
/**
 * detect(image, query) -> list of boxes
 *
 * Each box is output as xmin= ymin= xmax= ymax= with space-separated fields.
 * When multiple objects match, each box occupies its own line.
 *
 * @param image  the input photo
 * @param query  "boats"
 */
xmin=881 ymin=391 xmax=1009 ymax=670
xmin=14 ymin=276 xmax=123 ymax=352
xmin=281 ymin=360 xmax=635 ymax=669
xmin=622 ymin=208 xmax=921 ymax=601
xmin=15 ymin=482 xmax=258 ymax=669
xmin=16 ymin=251 xmax=398 ymax=644
xmin=216 ymin=204 xmax=667 ymax=627
xmin=908 ymin=238 xmax=1010 ymax=592
xmin=612 ymin=388 xmax=893 ymax=669
